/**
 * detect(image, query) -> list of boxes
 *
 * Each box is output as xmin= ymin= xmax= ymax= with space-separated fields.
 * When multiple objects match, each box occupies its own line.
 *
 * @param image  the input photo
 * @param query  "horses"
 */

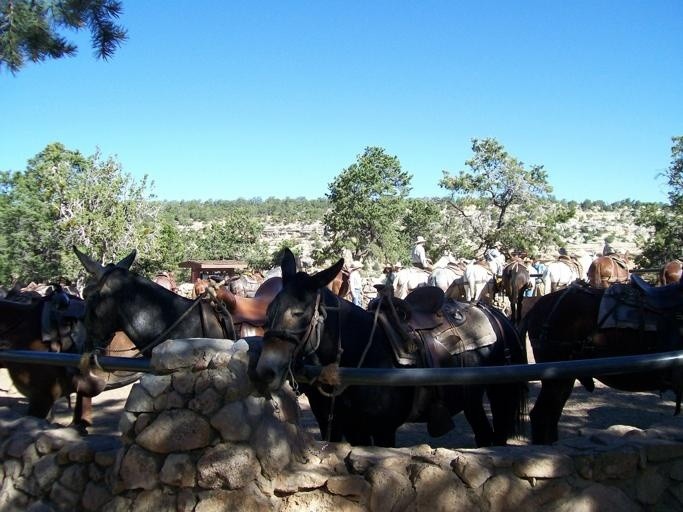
xmin=391 ymin=249 xmax=630 ymax=323
xmin=327 ymin=267 xmax=351 ymax=298
xmin=659 ymin=256 xmax=683 ymax=287
xmin=153 ymin=265 xmax=282 ymax=298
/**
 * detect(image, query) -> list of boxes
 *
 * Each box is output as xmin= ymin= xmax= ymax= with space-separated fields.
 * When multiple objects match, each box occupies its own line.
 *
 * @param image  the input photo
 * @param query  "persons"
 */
xmin=392 ymin=262 xmax=404 ymax=283
xmin=349 ymin=261 xmax=364 ymax=309
xmin=411 ymin=235 xmax=435 ymax=272
xmin=191 ymin=276 xmax=205 ymax=300
xmin=379 ymin=263 xmax=392 ymax=284
xmin=484 ymin=240 xmax=545 ymax=297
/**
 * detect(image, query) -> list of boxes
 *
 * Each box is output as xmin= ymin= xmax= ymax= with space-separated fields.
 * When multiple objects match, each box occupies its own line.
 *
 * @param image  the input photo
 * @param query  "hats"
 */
xmin=156 ymin=271 xmax=168 ymax=277
xmin=350 ymin=260 xmax=364 ymax=270
xmin=524 ymin=257 xmax=533 ymax=263
xmin=393 ymin=262 xmax=404 ymax=268
xmin=412 ymin=236 xmax=426 ymax=244
xmin=492 ymin=241 xmax=502 ymax=247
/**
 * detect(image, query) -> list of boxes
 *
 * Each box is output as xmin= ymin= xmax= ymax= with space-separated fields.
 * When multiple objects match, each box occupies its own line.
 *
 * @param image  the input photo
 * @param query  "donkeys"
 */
xmin=0 ymin=287 xmax=143 ymax=428
xmin=517 ymin=278 xmax=683 ymax=446
xmin=72 ymin=243 xmax=284 ymax=359
xmin=256 ymin=245 xmax=528 ymax=448
